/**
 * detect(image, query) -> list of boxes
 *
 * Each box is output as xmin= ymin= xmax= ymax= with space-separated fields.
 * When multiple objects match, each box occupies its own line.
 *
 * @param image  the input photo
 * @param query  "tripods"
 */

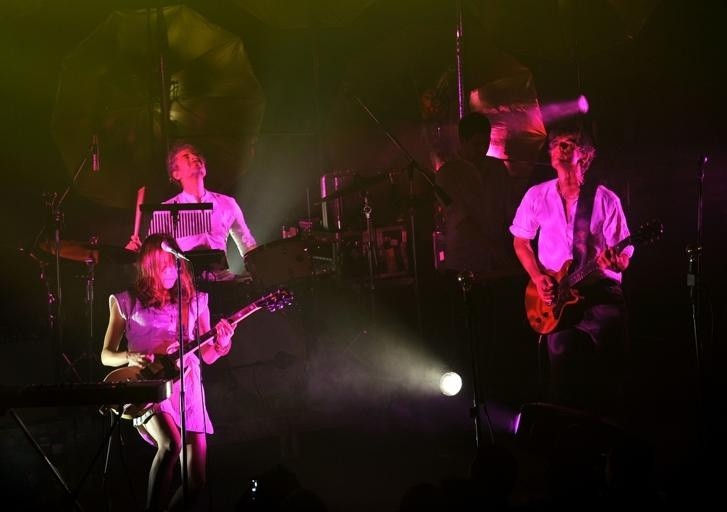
xmin=32 ymin=174 xmax=83 ymax=384
xmin=74 ymin=248 xmax=102 ymax=384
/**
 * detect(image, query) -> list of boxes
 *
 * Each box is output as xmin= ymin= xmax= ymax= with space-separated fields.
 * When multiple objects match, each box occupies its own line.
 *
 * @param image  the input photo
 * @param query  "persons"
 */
xmin=98 ymin=231 xmax=240 ymax=509
xmin=510 ymin=120 xmax=633 ymax=406
xmin=466 ymin=49 xmax=547 ymax=171
xmin=434 ymin=113 xmax=516 ymax=332
xmin=122 ymin=141 xmax=258 ymax=283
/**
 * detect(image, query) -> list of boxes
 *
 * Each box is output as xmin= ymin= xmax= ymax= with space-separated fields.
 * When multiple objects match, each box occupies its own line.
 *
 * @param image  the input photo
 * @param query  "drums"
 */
xmin=304 ymin=228 xmax=344 ymax=259
xmin=246 ymin=237 xmax=311 ymax=287
xmin=342 ymin=225 xmax=412 ymax=282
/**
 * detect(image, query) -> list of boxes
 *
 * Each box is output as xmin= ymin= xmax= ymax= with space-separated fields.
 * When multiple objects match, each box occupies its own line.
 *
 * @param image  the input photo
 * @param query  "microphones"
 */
xmin=684 ymin=241 xmax=703 ymax=288
xmin=92 ymin=136 xmax=100 ymax=172
xmin=457 ymin=268 xmax=476 ymax=317
xmin=160 ymin=240 xmax=190 ymax=262
xmin=429 ymin=183 xmax=452 ymax=206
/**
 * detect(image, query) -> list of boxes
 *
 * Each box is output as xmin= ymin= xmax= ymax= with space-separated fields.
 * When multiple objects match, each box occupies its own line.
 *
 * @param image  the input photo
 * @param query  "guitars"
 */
xmin=525 ymin=213 xmax=665 ymax=335
xmin=102 ymin=289 xmax=293 ymax=420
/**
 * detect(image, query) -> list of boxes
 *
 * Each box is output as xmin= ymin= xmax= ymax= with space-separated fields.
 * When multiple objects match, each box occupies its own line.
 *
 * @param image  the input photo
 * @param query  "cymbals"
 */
xmin=39 ymin=240 xmax=143 ymax=262
xmin=315 ymin=174 xmax=387 ymax=204
